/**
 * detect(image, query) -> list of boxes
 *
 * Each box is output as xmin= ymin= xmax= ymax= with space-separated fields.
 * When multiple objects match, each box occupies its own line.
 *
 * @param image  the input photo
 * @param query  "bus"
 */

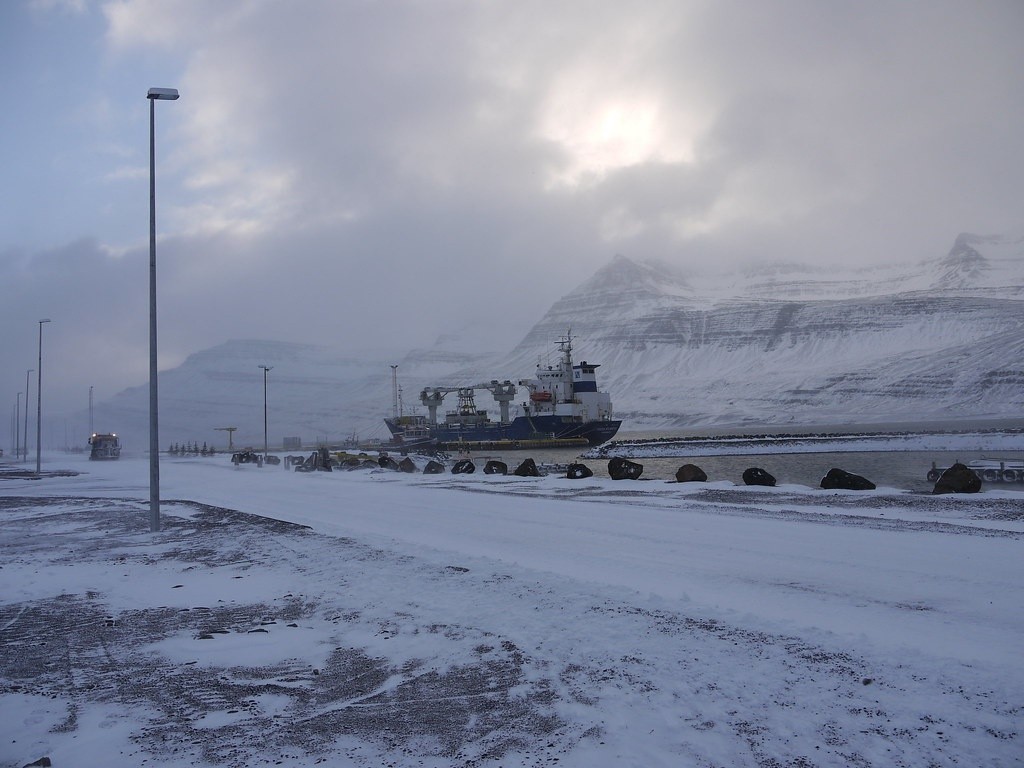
xmin=88 ymin=433 xmax=120 ymax=460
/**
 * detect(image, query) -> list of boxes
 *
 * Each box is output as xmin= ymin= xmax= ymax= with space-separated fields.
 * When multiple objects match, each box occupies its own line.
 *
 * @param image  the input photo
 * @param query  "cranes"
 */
xmin=214 ymin=427 xmax=237 ymax=450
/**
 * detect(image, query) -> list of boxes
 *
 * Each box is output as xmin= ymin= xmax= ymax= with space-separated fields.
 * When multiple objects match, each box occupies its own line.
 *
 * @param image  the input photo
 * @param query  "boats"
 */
xmin=385 ymin=330 xmax=621 ymax=451
xmin=360 ymin=438 xmax=380 ymax=451
xmin=377 ymin=424 xmax=439 ymax=453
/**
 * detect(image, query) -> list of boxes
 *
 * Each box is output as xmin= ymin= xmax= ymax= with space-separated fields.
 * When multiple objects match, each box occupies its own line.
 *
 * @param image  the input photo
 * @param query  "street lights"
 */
xmin=257 ymin=365 xmax=268 ymax=456
xmin=24 ymin=370 xmax=35 ymax=461
xmin=391 ymin=365 xmax=398 ymax=417
xmin=17 ymin=392 xmax=23 ymax=459
xmin=37 ymin=318 xmax=51 ymax=473
xmin=147 ymin=87 xmax=180 ymax=531
